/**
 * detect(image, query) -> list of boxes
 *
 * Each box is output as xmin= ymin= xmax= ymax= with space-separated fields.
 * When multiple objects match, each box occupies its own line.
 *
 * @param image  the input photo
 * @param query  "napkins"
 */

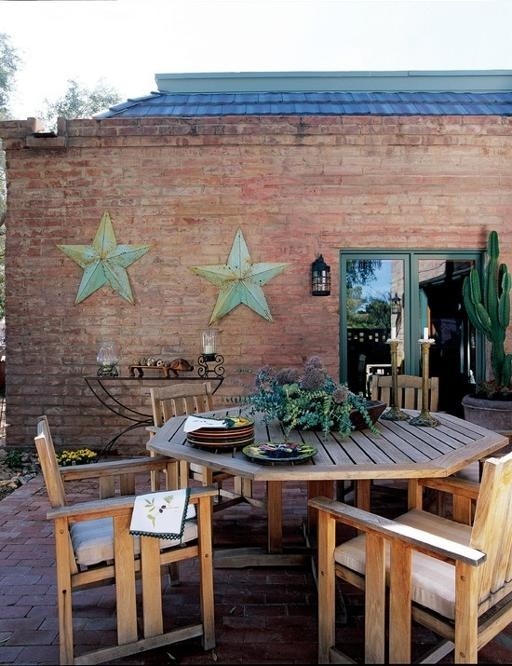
xmin=128 ymin=487 xmax=196 ymax=540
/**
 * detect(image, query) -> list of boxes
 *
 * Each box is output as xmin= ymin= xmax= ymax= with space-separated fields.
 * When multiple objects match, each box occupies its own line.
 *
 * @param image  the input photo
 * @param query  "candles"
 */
xmin=424 ymin=327 xmax=428 ymax=340
xmin=202 ymin=342 xmax=214 ymax=354
xmin=390 ymin=328 xmax=398 ymax=339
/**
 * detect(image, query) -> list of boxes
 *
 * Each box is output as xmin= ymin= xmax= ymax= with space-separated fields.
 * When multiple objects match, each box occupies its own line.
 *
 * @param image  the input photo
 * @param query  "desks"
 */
xmin=145 ymin=407 xmax=509 ymax=628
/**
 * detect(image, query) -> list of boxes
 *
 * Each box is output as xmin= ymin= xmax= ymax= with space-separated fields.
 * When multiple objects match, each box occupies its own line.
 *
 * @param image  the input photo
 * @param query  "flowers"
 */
xmin=243 ymin=357 xmax=381 ymax=443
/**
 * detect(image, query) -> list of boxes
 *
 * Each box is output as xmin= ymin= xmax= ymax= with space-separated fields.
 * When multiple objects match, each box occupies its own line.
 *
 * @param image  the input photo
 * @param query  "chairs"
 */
xmin=368 ymin=374 xmax=440 ymax=412
xmin=309 ymin=455 xmax=512 ymax=666
xmin=35 ymin=414 xmax=217 ymax=666
xmin=150 ymin=381 xmax=234 ymax=489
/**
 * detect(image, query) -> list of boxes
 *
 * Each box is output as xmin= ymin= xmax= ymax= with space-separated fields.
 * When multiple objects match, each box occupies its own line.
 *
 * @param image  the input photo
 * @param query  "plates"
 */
xmin=187 ymin=415 xmax=256 ymax=448
xmin=242 ymin=441 xmax=318 ymax=461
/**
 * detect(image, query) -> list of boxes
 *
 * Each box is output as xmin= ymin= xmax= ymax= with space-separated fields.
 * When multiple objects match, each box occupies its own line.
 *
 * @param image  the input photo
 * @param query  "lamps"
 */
xmin=311 ymin=253 xmax=332 ymax=297
xmin=388 ymin=293 xmax=401 ymax=314
xmin=96 ymin=341 xmax=121 ymax=376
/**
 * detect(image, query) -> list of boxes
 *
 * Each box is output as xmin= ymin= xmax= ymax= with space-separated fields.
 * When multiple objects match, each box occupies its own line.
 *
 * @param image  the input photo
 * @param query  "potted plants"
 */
xmin=460 ymin=231 xmax=512 ymax=453
